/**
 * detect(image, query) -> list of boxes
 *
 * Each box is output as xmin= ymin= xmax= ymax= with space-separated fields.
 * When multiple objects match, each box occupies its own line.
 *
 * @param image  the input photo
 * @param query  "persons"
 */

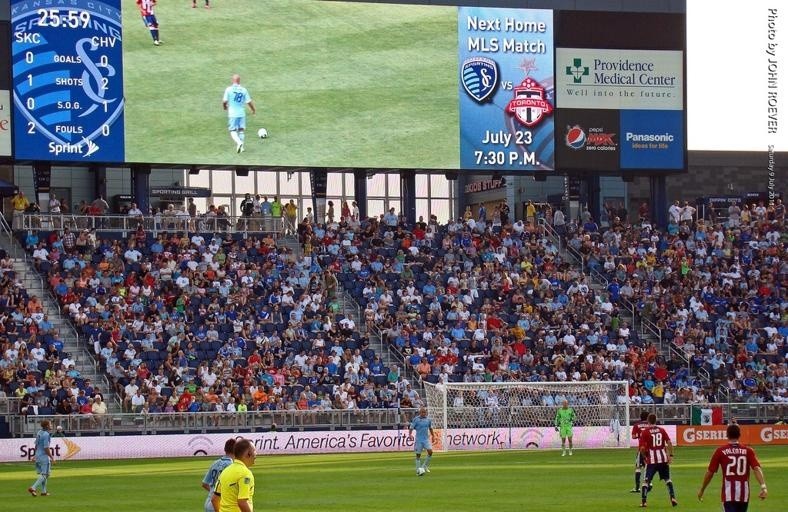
xmin=192 ymin=0 xmax=210 ymax=8
xmin=221 ymin=74 xmax=255 ymax=153
xmin=135 ymin=0 xmax=167 ymax=45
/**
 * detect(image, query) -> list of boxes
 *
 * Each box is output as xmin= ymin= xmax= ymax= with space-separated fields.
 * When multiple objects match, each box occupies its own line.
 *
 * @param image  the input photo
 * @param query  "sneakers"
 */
xmin=422 ymin=464 xmax=431 ymax=473
xmin=154 ymin=40 xmax=160 ymax=46
xmin=569 ymin=450 xmax=573 ymax=455
xmin=562 ymin=452 xmax=567 ymax=456
xmin=29 ymin=488 xmax=37 ymax=497
xmin=41 ymin=492 xmax=49 ymax=496
xmin=237 ymin=141 xmax=243 ymax=153
xmin=192 ymin=3 xmax=197 ymax=8
xmin=158 ymin=40 xmax=163 ymax=43
xmin=646 ymin=484 xmax=652 ymax=492
xmin=205 ymin=5 xmax=210 ymax=8
xmin=629 ymin=488 xmax=641 ymax=493
xmin=671 ymin=498 xmax=678 ymax=507
xmin=416 ymin=472 xmax=423 ymax=476
xmin=640 ymin=502 xmax=647 ymax=507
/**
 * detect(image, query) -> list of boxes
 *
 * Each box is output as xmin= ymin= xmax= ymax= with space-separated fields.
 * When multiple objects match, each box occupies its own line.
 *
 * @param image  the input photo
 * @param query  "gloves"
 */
xmin=555 ymin=426 xmax=559 ymax=431
xmin=667 ymin=456 xmax=674 ymax=466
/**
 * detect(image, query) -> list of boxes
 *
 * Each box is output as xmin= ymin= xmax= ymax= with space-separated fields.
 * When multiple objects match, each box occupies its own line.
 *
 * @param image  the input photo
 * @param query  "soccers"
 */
xmin=258 ymin=128 xmax=267 ymax=139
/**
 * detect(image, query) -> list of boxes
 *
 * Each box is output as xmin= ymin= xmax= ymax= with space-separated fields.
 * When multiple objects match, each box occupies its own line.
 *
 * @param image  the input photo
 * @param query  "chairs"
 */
xmin=0 ymin=206 xmax=788 ymax=416
